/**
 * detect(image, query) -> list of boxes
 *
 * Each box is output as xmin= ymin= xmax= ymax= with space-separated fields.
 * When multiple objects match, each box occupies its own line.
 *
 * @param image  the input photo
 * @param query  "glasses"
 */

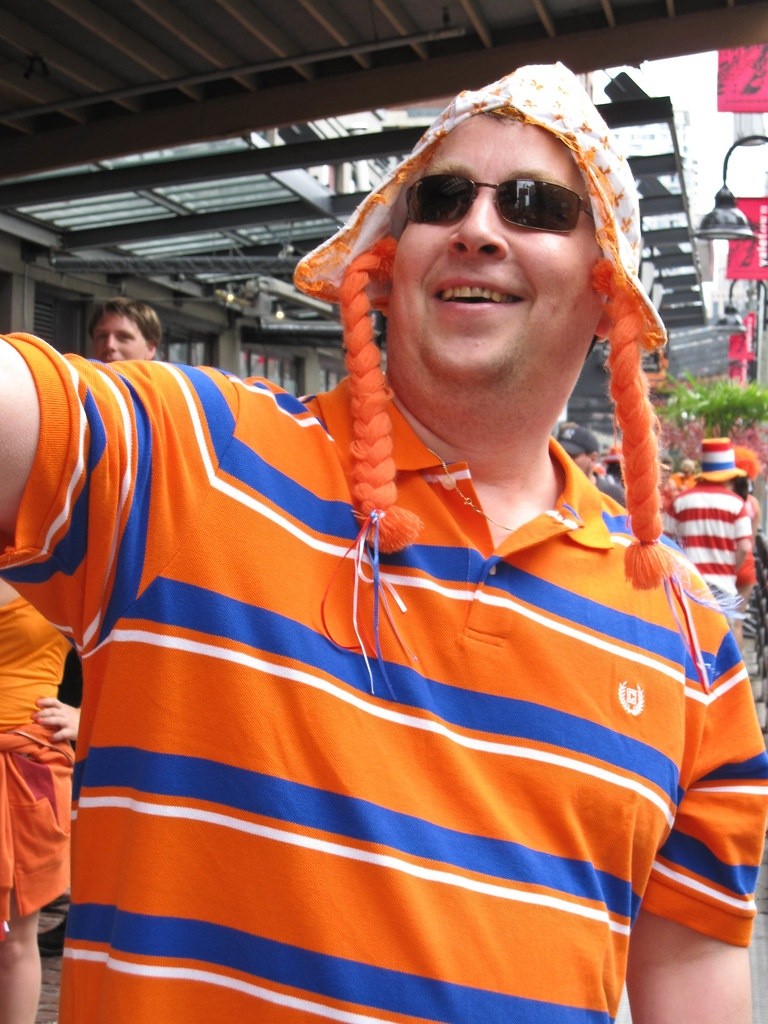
xmin=405 ymin=172 xmax=592 ymax=234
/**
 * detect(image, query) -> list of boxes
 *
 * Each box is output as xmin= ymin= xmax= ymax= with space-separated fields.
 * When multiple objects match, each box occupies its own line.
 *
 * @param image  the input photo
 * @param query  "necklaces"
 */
xmin=418 ymin=435 xmax=516 ymax=536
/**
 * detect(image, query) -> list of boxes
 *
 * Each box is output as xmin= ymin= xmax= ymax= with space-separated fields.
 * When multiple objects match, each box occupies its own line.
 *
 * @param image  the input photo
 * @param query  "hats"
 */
xmin=692 ymin=437 xmax=747 ymax=482
xmin=557 ymin=422 xmax=598 ymax=456
xmin=292 ymin=63 xmax=742 ymax=701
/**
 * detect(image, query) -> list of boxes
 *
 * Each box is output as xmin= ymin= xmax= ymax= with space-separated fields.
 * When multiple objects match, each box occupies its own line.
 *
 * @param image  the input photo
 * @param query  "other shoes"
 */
xmin=37 ymin=913 xmax=69 ymax=957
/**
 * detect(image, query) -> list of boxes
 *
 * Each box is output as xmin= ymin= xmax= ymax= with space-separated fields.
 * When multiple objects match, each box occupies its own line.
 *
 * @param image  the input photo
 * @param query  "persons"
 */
xmin=0 ymin=298 xmax=163 ymax=1024
xmin=0 ymin=60 xmax=768 ymax=1024
xmin=552 ymin=423 xmax=760 ymax=654
